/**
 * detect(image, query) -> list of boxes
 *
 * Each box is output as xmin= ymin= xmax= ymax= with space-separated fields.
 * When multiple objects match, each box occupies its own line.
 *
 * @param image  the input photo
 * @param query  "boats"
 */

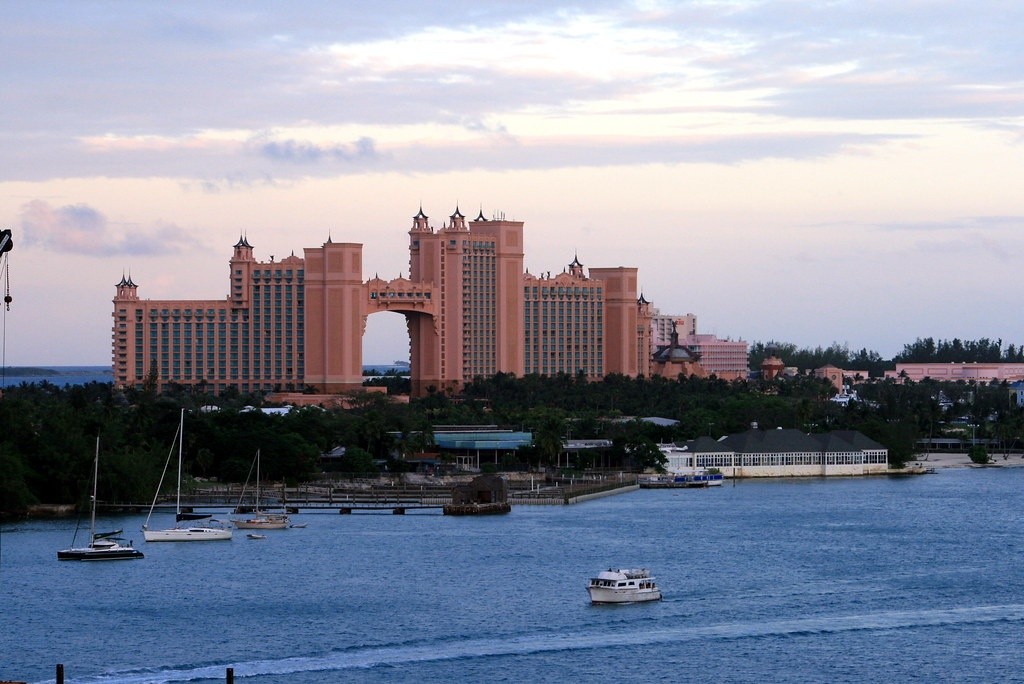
xmin=290 ymin=522 xmax=309 ymax=528
xmin=247 ymin=534 xmax=267 ymax=539
xmin=585 ymin=567 xmax=662 ymax=602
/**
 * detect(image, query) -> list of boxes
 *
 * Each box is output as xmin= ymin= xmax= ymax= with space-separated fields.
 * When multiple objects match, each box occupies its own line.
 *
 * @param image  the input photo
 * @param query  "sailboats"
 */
xmin=140 ymin=407 xmax=234 ymax=541
xmin=230 ymin=449 xmax=291 ymax=529
xmin=57 ymin=437 xmax=144 ymax=559
